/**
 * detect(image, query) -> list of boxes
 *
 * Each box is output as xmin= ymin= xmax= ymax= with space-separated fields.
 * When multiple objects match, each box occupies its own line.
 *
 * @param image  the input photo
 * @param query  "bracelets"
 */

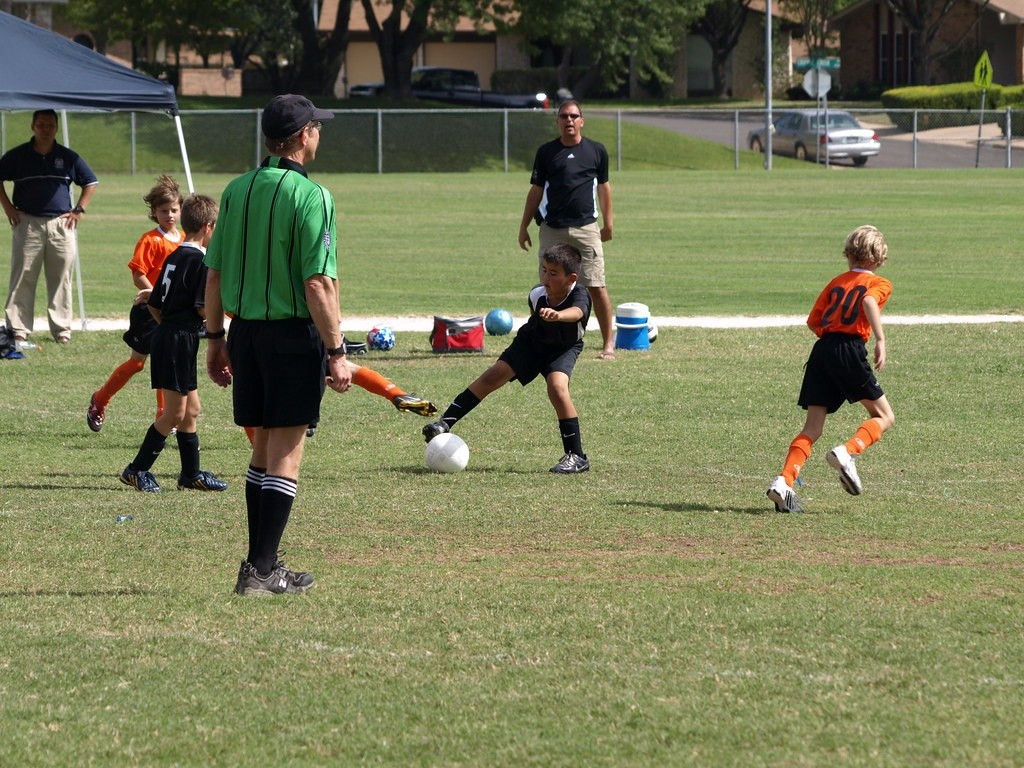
xmin=205 ymin=328 xmax=226 ymax=340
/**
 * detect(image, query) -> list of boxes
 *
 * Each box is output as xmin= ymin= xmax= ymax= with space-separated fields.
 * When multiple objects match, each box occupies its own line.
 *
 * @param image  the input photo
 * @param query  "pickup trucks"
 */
xmin=348 ymin=67 xmax=550 ymax=110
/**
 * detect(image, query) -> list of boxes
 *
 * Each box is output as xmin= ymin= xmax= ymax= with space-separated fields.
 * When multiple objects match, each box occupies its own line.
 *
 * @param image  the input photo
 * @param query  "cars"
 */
xmin=746 ymin=109 xmax=881 ymax=167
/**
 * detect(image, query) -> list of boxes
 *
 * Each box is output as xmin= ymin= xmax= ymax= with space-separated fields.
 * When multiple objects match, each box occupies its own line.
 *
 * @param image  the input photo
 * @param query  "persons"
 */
xmin=519 ymin=102 xmax=618 ymax=361
xmin=204 ymin=96 xmax=436 ymax=599
xmin=86 ymin=175 xmax=220 ymax=493
xmin=0 ymin=109 xmax=99 ymax=345
xmin=767 ymin=224 xmax=896 ymax=512
xmin=423 ymin=243 xmax=588 ymax=474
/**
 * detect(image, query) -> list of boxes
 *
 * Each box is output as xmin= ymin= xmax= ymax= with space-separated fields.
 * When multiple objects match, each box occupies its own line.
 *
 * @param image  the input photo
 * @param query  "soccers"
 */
xmin=484 ymin=308 xmax=513 ymax=336
xmin=424 ymin=432 xmax=470 ymax=473
xmin=647 ymin=317 xmax=658 ymax=344
xmin=365 ymin=325 xmax=397 ymax=352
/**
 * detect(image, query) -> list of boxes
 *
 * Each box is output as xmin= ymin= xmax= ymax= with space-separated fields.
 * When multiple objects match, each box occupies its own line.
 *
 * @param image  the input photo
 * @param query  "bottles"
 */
xmin=16 ymin=340 xmax=39 ymax=348
xmin=544 ymin=95 xmax=549 ymax=109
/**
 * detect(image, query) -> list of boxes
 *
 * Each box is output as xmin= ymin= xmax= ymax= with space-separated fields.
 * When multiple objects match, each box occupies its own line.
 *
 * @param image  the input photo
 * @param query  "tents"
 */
xmin=0 ymin=10 xmax=178 ymax=109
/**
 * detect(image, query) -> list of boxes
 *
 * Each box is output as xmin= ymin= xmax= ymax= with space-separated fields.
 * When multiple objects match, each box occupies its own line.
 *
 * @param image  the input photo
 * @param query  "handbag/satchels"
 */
xmin=428 ymin=315 xmax=484 ymax=353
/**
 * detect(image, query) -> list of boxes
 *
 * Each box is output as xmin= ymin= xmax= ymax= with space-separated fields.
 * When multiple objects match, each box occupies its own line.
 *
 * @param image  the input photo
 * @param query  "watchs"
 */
xmin=75 ymin=205 xmax=84 ymax=213
xmin=327 ymin=343 xmax=348 ymax=356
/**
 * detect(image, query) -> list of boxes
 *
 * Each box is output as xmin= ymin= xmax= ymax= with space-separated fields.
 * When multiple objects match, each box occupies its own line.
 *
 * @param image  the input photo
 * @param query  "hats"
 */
xmin=261 ymin=94 xmax=334 ymax=140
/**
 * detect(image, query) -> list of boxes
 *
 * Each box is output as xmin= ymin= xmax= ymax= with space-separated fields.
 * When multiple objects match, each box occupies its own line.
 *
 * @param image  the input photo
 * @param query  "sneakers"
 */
xmin=826 ymin=444 xmax=863 ymax=496
xmin=169 ymin=427 xmax=177 ymax=435
xmin=176 ymin=468 xmax=228 ymax=491
xmin=421 ymin=420 xmax=449 ymax=444
xmin=549 ymin=453 xmax=589 ymax=473
xmin=767 ymin=475 xmax=802 ymax=512
xmin=86 ymin=392 xmax=105 ymax=432
xmin=234 ymin=558 xmax=314 ymax=595
xmin=120 ymin=463 xmax=160 ymax=492
xmin=393 ymin=395 xmax=437 ymax=417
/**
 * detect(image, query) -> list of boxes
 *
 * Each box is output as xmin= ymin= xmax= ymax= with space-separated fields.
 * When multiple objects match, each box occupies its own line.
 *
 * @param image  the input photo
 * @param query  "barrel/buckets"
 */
xmin=614 ymin=302 xmax=650 ymax=351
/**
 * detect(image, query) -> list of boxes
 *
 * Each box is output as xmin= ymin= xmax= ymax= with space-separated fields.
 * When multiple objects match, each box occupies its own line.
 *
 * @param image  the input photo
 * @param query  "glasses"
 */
xmin=311 ymin=121 xmax=323 ymax=132
xmin=558 ymin=113 xmax=580 ymax=118
